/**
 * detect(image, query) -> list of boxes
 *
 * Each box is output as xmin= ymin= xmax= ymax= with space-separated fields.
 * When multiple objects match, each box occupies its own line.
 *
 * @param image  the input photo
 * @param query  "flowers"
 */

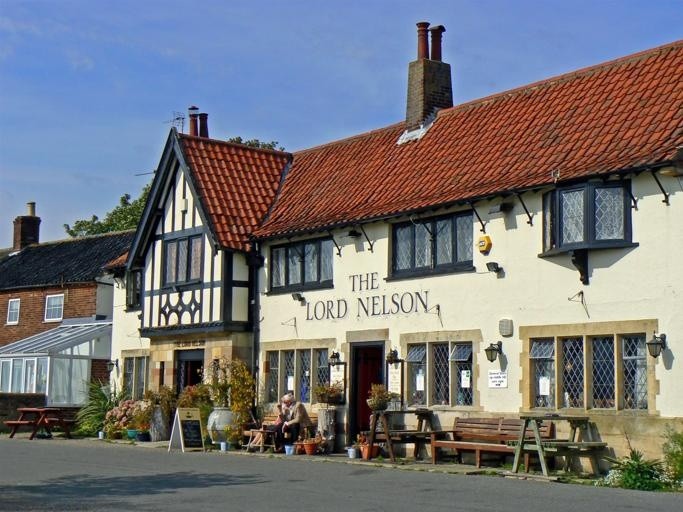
xmin=105 ymin=399 xmax=135 ymax=425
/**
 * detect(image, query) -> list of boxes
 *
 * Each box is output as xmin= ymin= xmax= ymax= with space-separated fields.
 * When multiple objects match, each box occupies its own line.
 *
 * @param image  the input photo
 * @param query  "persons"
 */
xmin=275 ymin=393 xmax=313 ymax=453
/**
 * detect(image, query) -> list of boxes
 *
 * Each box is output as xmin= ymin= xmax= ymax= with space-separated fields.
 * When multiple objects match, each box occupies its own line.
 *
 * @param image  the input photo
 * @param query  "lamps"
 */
xmin=330 ymin=351 xmax=339 ymax=366
xmin=645 ymin=330 xmax=666 ymax=359
xmin=484 ymin=341 xmax=502 ymax=362
xmin=106 ymin=358 xmax=118 ymax=371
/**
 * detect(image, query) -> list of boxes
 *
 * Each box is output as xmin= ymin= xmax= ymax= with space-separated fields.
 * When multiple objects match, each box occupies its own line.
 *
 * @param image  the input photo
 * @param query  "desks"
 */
xmin=366 ymin=409 xmax=447 ymax=461
xmin=512 ymin=416 xmax=602 ymax=478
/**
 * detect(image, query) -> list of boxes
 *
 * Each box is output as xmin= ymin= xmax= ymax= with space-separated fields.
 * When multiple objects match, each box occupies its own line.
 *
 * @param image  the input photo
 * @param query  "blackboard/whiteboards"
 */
xmin=176 ymin=407 xmax=205 ymax=451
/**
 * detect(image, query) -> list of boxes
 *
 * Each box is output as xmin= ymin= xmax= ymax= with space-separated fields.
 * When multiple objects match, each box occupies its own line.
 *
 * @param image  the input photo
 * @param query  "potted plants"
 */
xmin=367 ymin=383 xmax=398 ymax=410
xmin=177 ymin=355 xmax=255 ymax=444
xmin=313 ymin=380 xmax=344 ymax=404
xmin=143 ymin=386 xmax=176 ymax=442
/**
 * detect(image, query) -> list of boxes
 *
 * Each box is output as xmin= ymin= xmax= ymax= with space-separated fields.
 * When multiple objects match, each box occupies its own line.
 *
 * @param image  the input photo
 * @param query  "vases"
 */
xmin=293 ymin=437 xmax=321 ymax=455
xmin=360 ymin=443 xmax=377 ymax=460
xmin=348 ymin=447 xmax=356 ymax=458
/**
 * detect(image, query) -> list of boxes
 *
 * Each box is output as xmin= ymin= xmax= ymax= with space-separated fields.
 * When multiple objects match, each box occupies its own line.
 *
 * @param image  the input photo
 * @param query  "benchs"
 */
xmin=360 ymin=429 xmax=416 ymax=436
xmin=431 ymin=416 xmax=552 ymax=474
xmin=543 ymin=441 xmax=607 ymax=449
xmin=507 ymin=439 xmax=568 ymax=446
xmin=244 ymin=413 xmax=318 ymax=436
xmin=391 ymin=430 xmax=448 ymax=439
